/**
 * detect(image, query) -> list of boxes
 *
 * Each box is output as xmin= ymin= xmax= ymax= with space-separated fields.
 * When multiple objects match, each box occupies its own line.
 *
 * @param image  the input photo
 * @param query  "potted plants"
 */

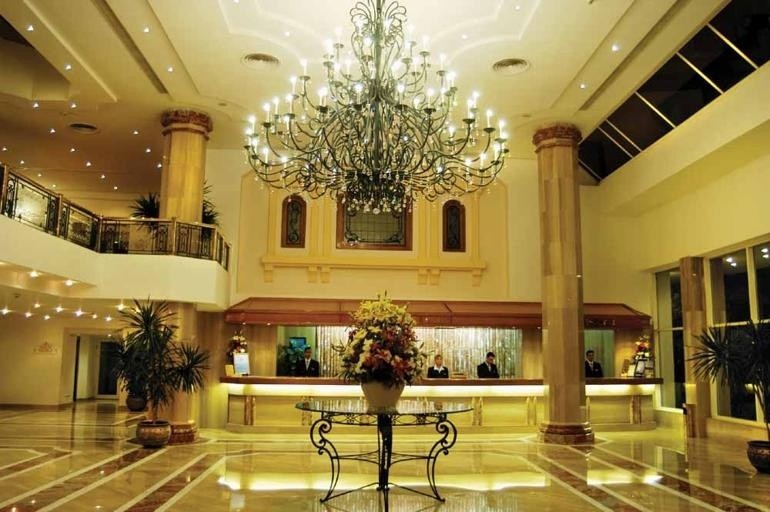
xmin=685 ymin=320 xmax=770 ymax=475
xmin=108 ymin=293 xmax=212 ymax=447
xmin=111 ymin=333 xmax=148 ymax=411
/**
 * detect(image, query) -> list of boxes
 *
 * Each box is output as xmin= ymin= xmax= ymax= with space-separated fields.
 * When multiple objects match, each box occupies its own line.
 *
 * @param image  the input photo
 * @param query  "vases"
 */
xmin=360 ymin=380 xmax=405 ymax=408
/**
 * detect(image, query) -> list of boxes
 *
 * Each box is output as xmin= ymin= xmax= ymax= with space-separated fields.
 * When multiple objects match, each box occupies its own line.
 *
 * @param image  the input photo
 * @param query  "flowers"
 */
xmin=227 ymin=331 xmax=247 ymax=357
xmin=330 ymin=289 xmax=434 ymax=388
xmin=632 ymin=337 xmax=651 ymax=359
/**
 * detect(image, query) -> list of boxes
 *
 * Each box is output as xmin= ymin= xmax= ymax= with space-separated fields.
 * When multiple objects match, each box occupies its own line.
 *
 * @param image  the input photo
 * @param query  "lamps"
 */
xmin=243 ymin=0 xmax=511 ymax=216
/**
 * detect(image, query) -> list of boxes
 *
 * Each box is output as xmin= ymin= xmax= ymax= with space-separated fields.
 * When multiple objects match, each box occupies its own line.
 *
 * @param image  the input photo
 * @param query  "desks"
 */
xmin=294 ymin=401 xmax=475 ymax=512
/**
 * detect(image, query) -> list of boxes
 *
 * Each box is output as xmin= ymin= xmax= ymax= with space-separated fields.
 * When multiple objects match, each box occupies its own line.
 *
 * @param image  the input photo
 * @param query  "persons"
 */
xmin=477 ymin=352 xmax=500 ymax=378
xmin=294 ymin=347 xmax=319 ymax=377
xmin=428 ymin=355 xmax=449 ymax=378
xmin=585 ymin=350 xmax=603 ymax=377
